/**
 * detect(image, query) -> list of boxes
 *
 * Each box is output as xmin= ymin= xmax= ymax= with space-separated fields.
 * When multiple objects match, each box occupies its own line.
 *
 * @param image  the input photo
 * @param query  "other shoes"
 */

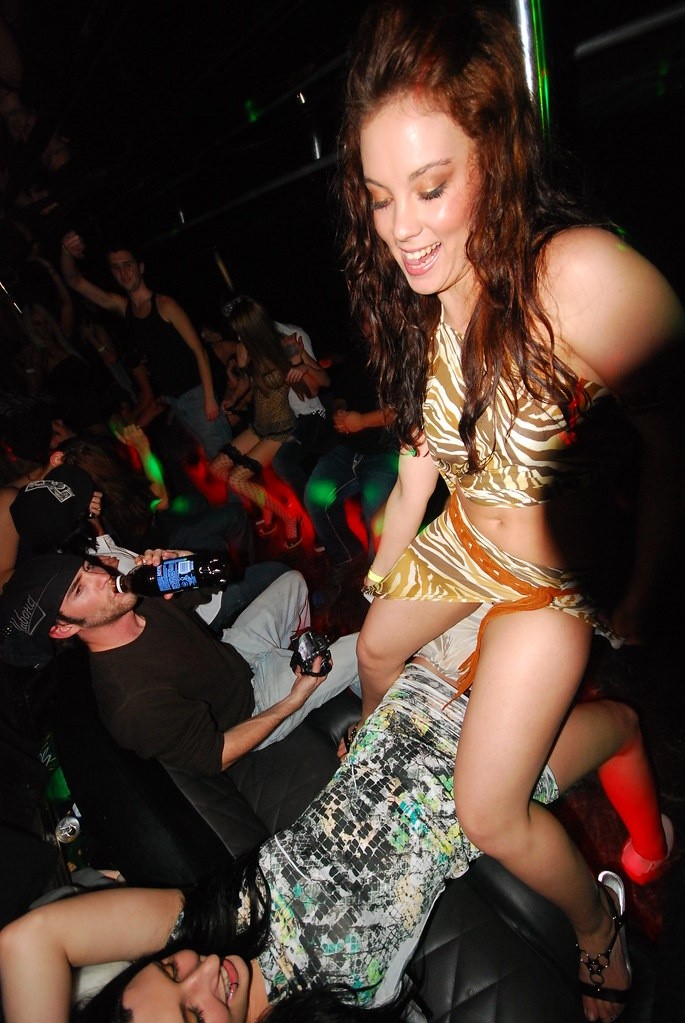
xmin=622 ymin=811 xmax=676 ymax=887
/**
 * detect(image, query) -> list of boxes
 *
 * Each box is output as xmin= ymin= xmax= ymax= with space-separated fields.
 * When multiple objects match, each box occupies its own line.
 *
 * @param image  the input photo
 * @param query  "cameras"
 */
xmin=289 ymin=627 xmax=331 ymax=673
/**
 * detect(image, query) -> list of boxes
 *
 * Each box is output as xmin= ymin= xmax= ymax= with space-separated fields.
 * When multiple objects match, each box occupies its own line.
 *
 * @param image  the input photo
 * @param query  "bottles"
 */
xmin=88 ymin=490 xmax=104 ymax=541
xmin=94 ymin=338 xmax=117 ymax=364
xmin=112 ymin=552 xmax=228 ymax=597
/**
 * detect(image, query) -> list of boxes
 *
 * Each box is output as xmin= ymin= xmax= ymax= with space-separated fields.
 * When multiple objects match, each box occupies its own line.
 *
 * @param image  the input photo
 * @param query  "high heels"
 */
xmin=282 ymin=518 xmax=303 ymax=549
xmin=572 ymin=869 xmax=636 ymax=1023
xmin=255 ymin=513 xmax=282 ymax=536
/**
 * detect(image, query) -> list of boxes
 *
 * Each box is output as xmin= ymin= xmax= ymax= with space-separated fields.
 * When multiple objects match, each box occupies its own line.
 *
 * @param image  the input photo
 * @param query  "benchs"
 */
xmin=50 ymin=681 xmax=588 ymax=1023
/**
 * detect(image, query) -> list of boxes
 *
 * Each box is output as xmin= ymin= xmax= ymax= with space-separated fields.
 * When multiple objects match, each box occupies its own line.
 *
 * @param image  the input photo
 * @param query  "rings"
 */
xmin=98 ymin=507 xmax=101 ymax=511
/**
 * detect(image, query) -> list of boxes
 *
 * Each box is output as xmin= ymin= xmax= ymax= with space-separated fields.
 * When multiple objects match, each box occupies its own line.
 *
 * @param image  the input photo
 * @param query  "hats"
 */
xmin=9 ymin=463 xmax=94 ymax=550
xmin=5 ymin=543 xmax=88 ymax=642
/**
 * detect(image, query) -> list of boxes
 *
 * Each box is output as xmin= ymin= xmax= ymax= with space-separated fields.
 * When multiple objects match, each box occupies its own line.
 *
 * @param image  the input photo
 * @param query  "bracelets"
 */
xmin=62 ymin=245 xmax=71 ymax=258
xmin=26 ymin=369 xmax=35 ymax=373
xmin=368 ymin=570 xmax=384 ymax=583
xmin=98 ymin=345 xmax=106 ymax=352
xmin=292 ymin=358 xmax=304 ymax=367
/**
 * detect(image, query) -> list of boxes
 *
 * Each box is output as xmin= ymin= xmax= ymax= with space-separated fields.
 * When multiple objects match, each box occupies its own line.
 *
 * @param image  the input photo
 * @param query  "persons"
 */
xmin=0 ymin=0 xmax=685 ymax=1023
xmin=336 ymin=1 xmax=681 ymax=1022
